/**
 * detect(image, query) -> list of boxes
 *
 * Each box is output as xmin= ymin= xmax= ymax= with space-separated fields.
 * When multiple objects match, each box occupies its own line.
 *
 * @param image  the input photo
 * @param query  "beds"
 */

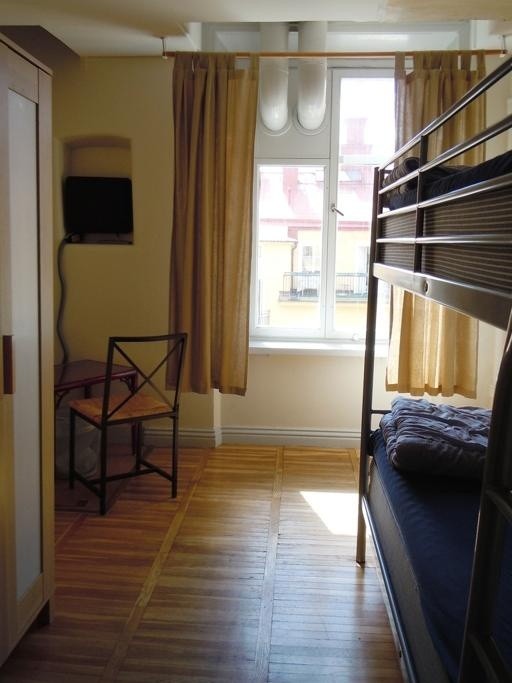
xmin=356 ymin=59 xmax=512 ymax=683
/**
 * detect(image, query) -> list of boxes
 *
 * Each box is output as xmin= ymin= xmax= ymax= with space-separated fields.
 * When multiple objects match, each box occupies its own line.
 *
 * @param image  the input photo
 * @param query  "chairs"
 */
xmin=67 ymin=333 xmax=187 ymax=515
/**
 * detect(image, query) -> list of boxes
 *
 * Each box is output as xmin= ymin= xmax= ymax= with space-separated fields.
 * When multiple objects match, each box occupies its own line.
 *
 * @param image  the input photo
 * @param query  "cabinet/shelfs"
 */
xmin=1 ymin=33 xmax=57 ymax=666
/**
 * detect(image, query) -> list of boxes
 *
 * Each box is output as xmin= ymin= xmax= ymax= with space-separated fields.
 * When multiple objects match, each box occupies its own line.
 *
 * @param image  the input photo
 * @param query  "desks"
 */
xmin=53 ymin=359 xmax=144 ymax=480
